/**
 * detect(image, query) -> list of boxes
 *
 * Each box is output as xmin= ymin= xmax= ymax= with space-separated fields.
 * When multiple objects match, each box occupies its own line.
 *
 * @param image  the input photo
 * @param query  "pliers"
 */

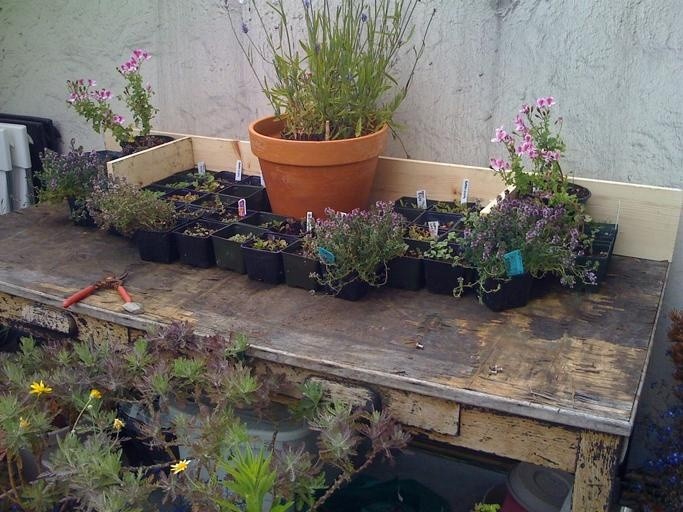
xmin=63 ymin=272 xmax=133 ymax=308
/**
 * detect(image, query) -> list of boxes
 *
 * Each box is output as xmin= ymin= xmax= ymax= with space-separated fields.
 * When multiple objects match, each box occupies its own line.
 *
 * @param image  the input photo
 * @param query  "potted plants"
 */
xmin=219 ymin=0 xmax=436 ymax=219
xmin=98 ymin=164 xmax=317 ymax=289
xmin=374 ymin=172 xmax=480 ymax=296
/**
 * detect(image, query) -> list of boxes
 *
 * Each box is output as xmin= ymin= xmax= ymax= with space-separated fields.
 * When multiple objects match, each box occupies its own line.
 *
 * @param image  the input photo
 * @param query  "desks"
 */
xmin=0 ymin=199 xmax=672 ymax=512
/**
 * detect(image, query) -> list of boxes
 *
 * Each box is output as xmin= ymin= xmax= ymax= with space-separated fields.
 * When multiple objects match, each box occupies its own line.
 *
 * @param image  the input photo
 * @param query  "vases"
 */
xmin=502 ymin=182 xmax=593 ymax=217
xmin=64 ymin=133 xmax=177 ymax=225
xmin=322 ymin=260 xmax=365 ymax=303
xmin=480 ymin=265 xmax=547 ymax=310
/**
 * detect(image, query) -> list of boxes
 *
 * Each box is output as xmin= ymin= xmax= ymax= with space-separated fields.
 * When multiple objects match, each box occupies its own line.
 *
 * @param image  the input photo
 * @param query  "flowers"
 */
xmin=488 ymin=93 xmax=582 ymax=207
xmin=30 ymin=48 xmax=169 ymax=234
xmin=297 ymin=196 xmax=406 ymax=290
xmin=447 ymin=194 xmax=601 ymax=292
